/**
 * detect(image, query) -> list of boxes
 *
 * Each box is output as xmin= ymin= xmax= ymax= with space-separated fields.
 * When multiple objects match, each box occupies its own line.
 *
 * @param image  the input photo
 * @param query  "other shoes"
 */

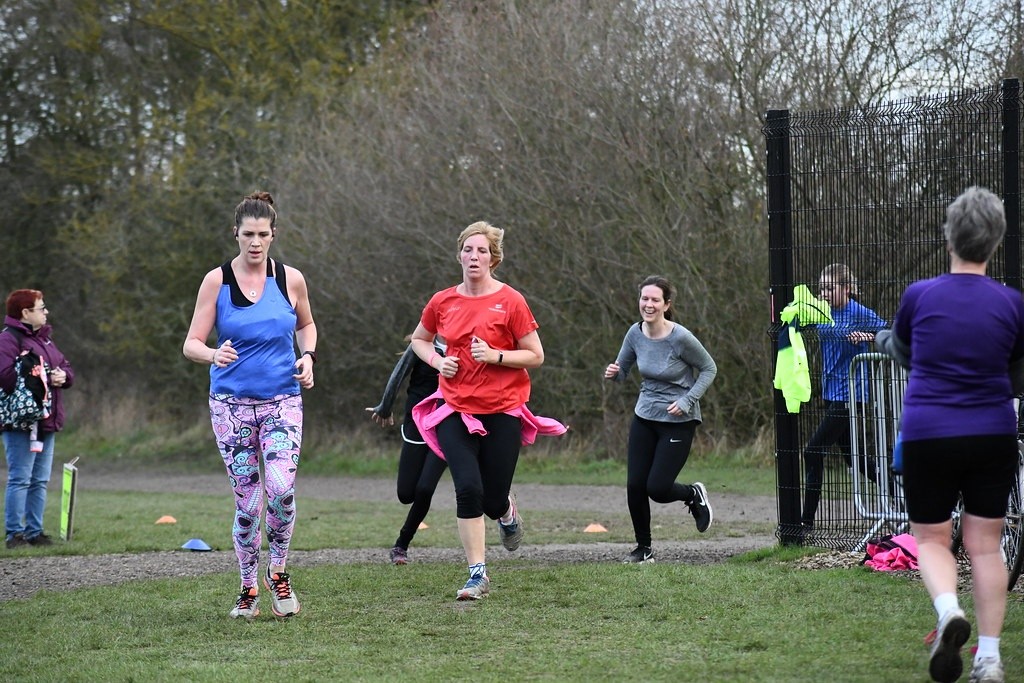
xmin=6 ymin=532 xmax=30 ymax=549
xmin=27 ymin=532 xmax=53 ymax=547
xmin=801 ymin=525 xmax=815 ymax=545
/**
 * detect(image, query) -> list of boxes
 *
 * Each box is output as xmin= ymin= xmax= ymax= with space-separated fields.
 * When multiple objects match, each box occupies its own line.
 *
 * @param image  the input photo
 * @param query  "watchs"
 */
xmin=497 ymin=351 xmax=504 ymax=365
xmin=303 ymin=351 xmax=317 ymax=363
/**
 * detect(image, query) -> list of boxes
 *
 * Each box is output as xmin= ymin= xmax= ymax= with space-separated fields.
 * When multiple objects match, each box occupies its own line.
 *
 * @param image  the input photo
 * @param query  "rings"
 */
xmin=474 ymin=353 xmax=478 ymax=358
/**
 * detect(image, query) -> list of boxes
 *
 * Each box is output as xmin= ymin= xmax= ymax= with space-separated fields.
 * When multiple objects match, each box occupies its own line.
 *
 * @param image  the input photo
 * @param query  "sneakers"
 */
xmin=684 ymin=482 xmax=713 ymax=533
xmin=622 ymin=546 xmax=654 ymax=565
xmin=456 ymin=573 xmax=490 ymax=599
xmin=389 ymin=547 xmax=407 ymax=565
xmin=968 ymin=645 xmax=1004 ymax=683
xmin=263 ymin=565 xmax=300 ymax=616
xmin=230 ymin=586 xmax=259 ymax=619
xmin=498 ymin=490 xmax=524 ymax=551
xmin=924 ymin=608 xmax=971 ymax=683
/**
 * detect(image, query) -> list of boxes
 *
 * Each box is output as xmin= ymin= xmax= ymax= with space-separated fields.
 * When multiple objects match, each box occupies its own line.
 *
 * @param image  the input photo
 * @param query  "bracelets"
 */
xmin=213 ymin=349 xmax=217 ymax=363
xmin=429 ymin=352 xmax=440 ymax=366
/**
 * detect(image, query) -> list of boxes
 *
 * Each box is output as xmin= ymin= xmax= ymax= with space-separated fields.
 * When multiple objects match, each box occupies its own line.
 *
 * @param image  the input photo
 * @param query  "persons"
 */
xmin=412 ymin=221 xmax=544 ymax=598
xmin=0 ymin=289 xmax=74 ymax=549
xmin=366 ymin=330 xmax=448 ymax=566
xmin=184 ymin=192 xmax=317 ymax=613
xmin=605 ymin=276 xmax=717 ymax=564
xmin=894 ymin=188 xmax=1024 ymax=683
xmin=802 ymin=264 xmax=894 ymax=523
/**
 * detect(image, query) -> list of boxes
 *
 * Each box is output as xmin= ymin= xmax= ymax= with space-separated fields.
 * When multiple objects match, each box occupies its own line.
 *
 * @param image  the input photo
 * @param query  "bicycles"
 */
xmin=949 ymin=430 xmax=1024 ymax=592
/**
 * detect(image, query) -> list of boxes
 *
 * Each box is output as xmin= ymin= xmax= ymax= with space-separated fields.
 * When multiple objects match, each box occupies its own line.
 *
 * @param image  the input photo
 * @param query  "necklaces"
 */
xmin=250 ymin=291 xmax=256 ymax=296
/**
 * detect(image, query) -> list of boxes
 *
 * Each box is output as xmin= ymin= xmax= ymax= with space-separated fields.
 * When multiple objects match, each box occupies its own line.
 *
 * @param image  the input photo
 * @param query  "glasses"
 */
xmin=28 ymin=305 xmax=46 ymax=311
